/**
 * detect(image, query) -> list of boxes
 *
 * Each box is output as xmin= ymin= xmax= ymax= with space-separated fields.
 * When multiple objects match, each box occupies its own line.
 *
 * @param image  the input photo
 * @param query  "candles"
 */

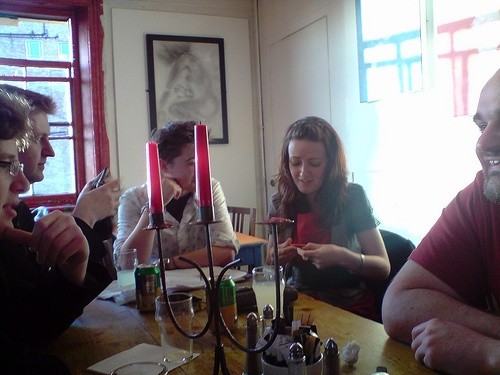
xmin=147 ymin=142 xmax=164 ymax=214
xmin=193 ymin=122 xmax=212 ymax=207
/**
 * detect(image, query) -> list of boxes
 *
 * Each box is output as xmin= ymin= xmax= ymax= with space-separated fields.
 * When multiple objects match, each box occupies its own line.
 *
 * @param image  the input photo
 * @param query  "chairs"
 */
xmin=362 ymin=229 xmax=415 ymax=325
xmin=227 ymin=206 xmax=256 ymax=237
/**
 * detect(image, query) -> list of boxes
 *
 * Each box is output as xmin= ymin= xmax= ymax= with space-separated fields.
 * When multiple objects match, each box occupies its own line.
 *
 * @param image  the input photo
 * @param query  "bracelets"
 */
xmin=349 ymin=254 xmax=365 ymax=275
xmin=141 ymin=201 xmax=149 ymax=213
xmin=171 ymin=257 xmax=176 ymax=267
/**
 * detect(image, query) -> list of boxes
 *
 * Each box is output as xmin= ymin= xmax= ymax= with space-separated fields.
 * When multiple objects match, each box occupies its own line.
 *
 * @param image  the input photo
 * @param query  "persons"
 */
xmin=113 ymin=120 xmax=238 ymax=271
xmin=265 ymin=118 xmax=391 ymax=319
xmin=0 ymin=84 xmax=120 ymax=375
xmin=382 ymin=68 xmax=500 ymax=375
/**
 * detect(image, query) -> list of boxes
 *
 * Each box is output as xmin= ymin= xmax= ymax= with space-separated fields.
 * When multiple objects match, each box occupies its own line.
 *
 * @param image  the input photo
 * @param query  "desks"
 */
xmin=45 ymin=265 xmax=451 ymax=375
xmin=234 ymin=232 xmax=265 ymax=268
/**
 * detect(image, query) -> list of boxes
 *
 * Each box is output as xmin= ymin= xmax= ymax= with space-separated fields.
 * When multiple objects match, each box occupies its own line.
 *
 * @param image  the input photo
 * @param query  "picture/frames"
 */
xmin=146 ymin=34 xmax=229 ymax=145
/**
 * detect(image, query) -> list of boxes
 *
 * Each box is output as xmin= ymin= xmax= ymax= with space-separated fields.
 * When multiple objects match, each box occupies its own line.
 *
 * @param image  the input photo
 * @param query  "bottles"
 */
xmin=242 ymin=311 xmax=265 ymax=375
xmin=287 ymin=342 xmax=308 ymax=375
xmin=262 ymin=303 xmax=274 ymax=338
xmin=322 ymin=337 xmax=340 ymax=375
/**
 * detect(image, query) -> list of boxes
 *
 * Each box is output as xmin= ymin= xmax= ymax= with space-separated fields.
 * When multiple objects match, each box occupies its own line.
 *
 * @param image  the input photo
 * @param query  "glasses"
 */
xmin=0 ymin=160 xmax=24 ymax=176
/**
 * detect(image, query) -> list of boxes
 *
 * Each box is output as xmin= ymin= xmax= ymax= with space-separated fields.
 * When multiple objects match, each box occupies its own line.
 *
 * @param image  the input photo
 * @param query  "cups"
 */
xmin=252 ymin=264 xmax=285 ymax=321
xmin=113 ymin=248 xmax=138 ymax=289
xmin=154 ymin=292 xmax=194 ymax=363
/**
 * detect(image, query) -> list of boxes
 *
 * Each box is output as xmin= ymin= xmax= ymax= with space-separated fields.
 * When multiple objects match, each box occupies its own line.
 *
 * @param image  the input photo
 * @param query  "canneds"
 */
xmin=205 ymin=275 xmax=237 ymax=334
xmin=134 ymin=263 xmax=162 ymax=312
